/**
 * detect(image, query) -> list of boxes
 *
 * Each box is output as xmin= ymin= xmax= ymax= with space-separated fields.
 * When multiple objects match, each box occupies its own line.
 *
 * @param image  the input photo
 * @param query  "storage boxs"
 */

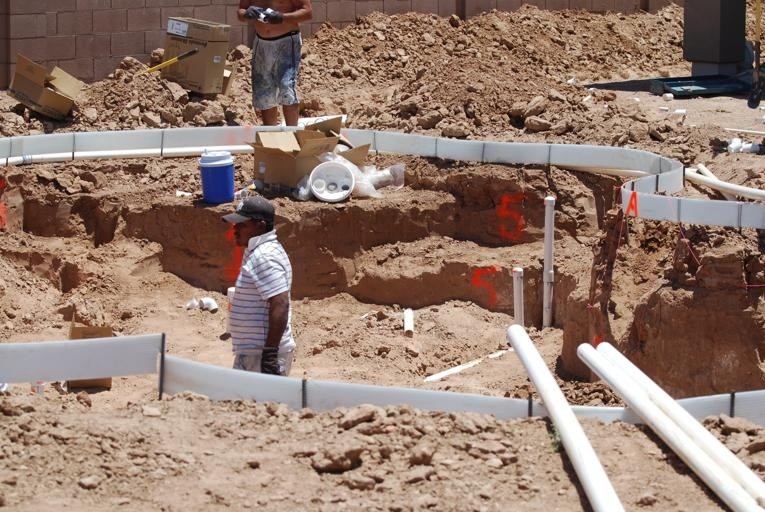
xmin=159 ymin=15 xmax=240 ymax=105
xmin=6 ymin=51 xmax=84 ymax=121
xmin=243 ymin=116 xmax=372 ymax=196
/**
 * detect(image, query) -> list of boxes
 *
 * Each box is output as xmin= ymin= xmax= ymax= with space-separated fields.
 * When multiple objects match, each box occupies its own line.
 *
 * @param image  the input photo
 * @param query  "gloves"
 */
xmin=261 ymin=348 xmax=279 ymax=375
xmin=243 ymin=6 xmax=282 ymax=23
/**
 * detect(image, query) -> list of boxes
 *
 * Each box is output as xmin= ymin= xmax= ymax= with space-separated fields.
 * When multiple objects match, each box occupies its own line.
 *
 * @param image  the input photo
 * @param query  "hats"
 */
xmin=219 ymin=196 xmax=275 ymax=223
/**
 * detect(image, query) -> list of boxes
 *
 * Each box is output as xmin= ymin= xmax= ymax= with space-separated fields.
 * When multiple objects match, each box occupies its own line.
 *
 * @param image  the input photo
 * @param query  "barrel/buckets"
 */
xmin=197 ymin=152 xmax=235 ymax=203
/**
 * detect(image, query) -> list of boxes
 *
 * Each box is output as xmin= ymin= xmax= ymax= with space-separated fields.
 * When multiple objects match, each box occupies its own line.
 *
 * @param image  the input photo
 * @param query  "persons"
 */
xmin=222 ymin=196 xmax=297 ymax=377
xmin=237 ymin=0 xmax=313 ymax=126
